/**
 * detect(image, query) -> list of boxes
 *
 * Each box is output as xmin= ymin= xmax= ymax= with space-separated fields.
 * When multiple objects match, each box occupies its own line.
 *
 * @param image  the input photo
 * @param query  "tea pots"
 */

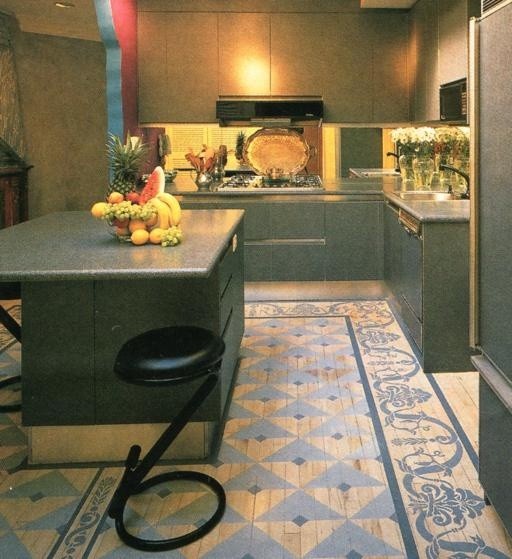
xmin=189 ymin=168 xmax=214 ymax=191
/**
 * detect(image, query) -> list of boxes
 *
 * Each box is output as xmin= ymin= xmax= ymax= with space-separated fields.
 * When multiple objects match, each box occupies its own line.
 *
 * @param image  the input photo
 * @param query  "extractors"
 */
xmin=215 ymin=95 xmax=324 ymax=128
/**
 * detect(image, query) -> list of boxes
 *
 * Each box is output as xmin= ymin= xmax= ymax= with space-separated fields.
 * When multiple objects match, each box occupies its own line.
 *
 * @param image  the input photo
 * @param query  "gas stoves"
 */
xmin=218 ymin=174 xmax=323 ymax=189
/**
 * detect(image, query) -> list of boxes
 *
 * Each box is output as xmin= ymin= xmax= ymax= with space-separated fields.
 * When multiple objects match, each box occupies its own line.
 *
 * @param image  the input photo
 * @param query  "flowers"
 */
xmin=412 ymin=127 xmax=437 ymax=155
xmin=393 ymin=129 xmax=414 ymax=155
xmin=431 ymin=127 xmax=459 ymax=153
xmin=452 ymin=128 xmax=469 ymax=156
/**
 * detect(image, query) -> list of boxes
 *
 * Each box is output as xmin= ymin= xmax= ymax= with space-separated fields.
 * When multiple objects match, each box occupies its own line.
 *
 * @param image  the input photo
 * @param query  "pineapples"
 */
xmin=102 ymin=129 xmax=155 ymax=203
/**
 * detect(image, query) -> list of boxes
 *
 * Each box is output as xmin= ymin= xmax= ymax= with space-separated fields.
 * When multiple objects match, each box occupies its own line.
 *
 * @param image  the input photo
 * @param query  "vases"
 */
xmin=452 ymin=158 xmax=471 ymax=195
xmin=435 ymin=153 xmax=451 ymax=191
xmin=411 ymin=157 xmax=434 ymax=189
xmin=398 ymin=156 xmax=413 ymax=181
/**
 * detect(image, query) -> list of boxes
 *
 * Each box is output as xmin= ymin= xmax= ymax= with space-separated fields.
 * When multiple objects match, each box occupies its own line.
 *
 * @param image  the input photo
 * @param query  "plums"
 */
xmin=127 ymin=191 xmax=140 ymax=204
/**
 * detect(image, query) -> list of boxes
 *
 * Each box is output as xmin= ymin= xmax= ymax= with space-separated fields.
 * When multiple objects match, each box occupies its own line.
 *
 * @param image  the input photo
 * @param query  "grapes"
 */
xmin=100 ymin=202 xmax=154 ymax=222
xmin=162 ymin=226 xmax=184 ymax=248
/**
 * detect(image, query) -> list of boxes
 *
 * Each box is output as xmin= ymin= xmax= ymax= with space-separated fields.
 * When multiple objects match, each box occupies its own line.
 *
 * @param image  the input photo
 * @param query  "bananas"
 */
xmin=148 ymin=192 xmax=182 ymax=230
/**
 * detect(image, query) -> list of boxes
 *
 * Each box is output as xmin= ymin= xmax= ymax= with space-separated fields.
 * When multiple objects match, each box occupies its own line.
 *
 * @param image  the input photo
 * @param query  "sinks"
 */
xmin=360 ymin=171 xmax=401 ymax=177
xmin=392 ymin=190 xmax=470 ymax=201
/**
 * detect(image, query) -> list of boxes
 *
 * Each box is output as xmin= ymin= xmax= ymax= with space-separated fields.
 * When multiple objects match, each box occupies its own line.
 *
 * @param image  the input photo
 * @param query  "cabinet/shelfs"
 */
xmin=237 ymin=204 xmax=327 ymax=281
xmin=385 ymin=203 xmax=398 ymax=315
xmin=326 ymin=200 xmax=384 ymax=285
xmin=139 ymin=6 xmax=217 ymax=125
xmin=217 ymin=10 xmax=317 ymax=98
xmin=317 ymin=8 xmax=407 ymax=124
xmin=405 ymin=0 xmax=470 ymax=125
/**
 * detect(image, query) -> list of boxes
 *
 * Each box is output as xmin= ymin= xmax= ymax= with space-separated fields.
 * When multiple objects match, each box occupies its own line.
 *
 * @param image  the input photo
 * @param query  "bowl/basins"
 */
xmin=164 ymin=171 xmax=177 ymax=183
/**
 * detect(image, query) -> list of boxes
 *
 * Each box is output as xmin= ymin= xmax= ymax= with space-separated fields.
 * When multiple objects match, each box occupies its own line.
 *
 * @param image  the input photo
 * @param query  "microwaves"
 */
xmin=438 ymin=77 xmax=468 ymax=124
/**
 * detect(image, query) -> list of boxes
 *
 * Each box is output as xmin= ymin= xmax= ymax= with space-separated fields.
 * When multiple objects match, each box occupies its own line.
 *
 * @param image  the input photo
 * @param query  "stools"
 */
xmin=108 ymin=326 xmax=230 ymax=553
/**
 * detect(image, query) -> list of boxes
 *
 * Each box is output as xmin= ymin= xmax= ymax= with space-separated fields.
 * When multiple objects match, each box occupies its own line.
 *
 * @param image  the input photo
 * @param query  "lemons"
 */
xmin=108 ymin=191 xmax=124 ymax=204
xmin=128 ymin=220 xmax=147 ymax=235
xmin=130 ymin=228 xmax=149 ymax=246
xmin=149 ymin=228 xmax=164 ymax=244
xmin=112 ymin=217 xmax=130 ymax=236
xmin=90 ymin=202 xmax=109 ymax=218
xmin=145 ymin=214 xmax=158 ymax=229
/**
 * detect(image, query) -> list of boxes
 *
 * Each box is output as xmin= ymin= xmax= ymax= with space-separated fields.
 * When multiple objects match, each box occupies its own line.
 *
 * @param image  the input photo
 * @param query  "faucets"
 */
xmin=439 ymin=164 xmax=470 ymax=199
xmin=386 ymin=152 xmax=401 ymax=172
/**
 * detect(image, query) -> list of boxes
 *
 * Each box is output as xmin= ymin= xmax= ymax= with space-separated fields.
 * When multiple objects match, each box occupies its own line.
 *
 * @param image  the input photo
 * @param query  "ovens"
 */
xmin=395 ymin=206 xmax=423 ymax=368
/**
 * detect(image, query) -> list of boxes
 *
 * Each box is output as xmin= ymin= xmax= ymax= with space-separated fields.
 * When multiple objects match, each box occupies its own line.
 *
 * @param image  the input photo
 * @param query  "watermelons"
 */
xmin=139 ymin=165 xmax=165 ymax=205
